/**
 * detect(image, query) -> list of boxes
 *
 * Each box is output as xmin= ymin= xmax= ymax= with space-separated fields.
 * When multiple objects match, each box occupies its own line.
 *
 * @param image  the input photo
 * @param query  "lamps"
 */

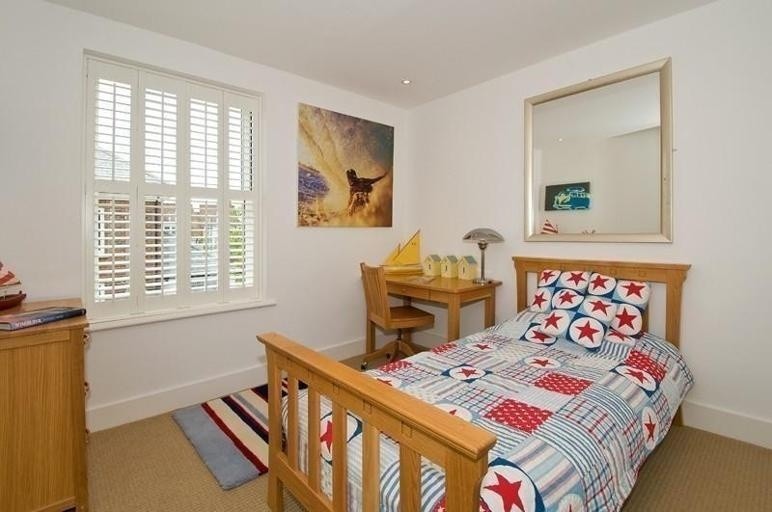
xmin=461 ymin=228 xmax=506 ymax=284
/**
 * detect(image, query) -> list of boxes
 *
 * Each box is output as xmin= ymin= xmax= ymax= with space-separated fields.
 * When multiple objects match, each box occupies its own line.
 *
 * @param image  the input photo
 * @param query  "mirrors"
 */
xmin=522 ymin=57 xmax=674 ymax=243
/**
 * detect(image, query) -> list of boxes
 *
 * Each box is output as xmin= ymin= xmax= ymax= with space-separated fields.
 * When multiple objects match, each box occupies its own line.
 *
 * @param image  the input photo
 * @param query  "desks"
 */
xmin=361 ymin=272 xmax=503 ymax=353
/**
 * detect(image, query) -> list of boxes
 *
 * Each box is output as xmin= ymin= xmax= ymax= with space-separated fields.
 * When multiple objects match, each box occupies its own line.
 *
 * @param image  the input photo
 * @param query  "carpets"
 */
xmin=169 ymin=377 xmax=308 ymax=492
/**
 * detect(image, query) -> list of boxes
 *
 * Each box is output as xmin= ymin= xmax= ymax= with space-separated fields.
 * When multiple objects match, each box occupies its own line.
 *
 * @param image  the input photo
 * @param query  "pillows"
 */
xmin=527 ymin=269 xmax=652 ymax=354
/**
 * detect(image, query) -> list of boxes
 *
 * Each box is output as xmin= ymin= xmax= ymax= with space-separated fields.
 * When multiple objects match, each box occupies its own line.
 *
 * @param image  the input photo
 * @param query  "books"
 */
xmin=0 ymin=306 xmax=86 ymax=331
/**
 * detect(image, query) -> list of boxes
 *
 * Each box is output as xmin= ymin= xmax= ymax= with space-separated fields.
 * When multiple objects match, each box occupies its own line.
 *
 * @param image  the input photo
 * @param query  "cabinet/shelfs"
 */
xmin=0 ymin=298 xmax=91 ymax=511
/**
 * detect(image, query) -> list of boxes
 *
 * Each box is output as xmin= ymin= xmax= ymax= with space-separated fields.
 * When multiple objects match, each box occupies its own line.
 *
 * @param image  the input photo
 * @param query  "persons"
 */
xmin=344 ymin=167 xmax=391 ymax=218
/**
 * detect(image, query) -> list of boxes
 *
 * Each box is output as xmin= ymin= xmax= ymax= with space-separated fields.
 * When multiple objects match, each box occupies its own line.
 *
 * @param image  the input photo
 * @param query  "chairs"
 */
xmin=360 ymin=263 xmax=435 ymax=370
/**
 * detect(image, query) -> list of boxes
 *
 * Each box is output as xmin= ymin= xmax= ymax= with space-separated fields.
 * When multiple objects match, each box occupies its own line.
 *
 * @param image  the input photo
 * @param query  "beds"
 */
xmin=255 ymin=256 xmax=693 ymax=512
